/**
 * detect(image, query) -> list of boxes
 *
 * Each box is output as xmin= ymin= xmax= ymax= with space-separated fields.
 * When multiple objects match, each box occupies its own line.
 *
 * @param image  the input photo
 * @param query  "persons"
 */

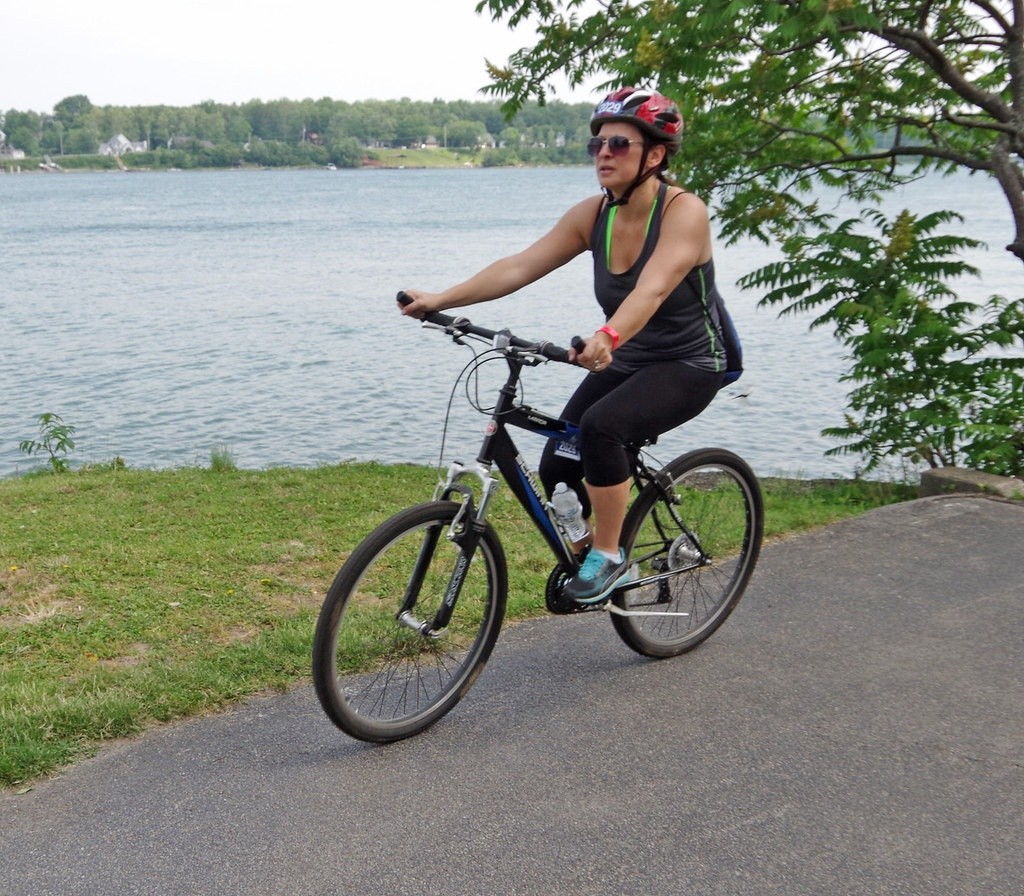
xmin=397 ymin=88 xmax=728 ymax=603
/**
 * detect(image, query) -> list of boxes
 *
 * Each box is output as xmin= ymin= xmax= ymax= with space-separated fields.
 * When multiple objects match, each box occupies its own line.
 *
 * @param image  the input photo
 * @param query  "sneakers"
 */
xmin=563 ymin=546 xmax=629 ymax=603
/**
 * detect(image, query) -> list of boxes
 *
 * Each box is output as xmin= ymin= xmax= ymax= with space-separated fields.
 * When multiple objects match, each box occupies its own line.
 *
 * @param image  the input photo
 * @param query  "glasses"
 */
xmin=587 ymin=136 xmax=644 ymax=156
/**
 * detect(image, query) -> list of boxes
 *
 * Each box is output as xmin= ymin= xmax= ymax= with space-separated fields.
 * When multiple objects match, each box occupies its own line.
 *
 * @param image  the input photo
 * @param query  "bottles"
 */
xmin=551 ymin=482 xmax=586 ymax=543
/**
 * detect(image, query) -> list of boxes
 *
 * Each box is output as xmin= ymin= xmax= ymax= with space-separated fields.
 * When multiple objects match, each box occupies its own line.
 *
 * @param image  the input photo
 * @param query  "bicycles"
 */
xmin=312 ymin=290 xmax=768 ymax=744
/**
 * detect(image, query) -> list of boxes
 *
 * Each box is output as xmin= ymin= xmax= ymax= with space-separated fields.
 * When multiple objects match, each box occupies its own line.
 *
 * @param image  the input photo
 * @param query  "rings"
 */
xmin=595 ymin=360 xmax=600 ymax=367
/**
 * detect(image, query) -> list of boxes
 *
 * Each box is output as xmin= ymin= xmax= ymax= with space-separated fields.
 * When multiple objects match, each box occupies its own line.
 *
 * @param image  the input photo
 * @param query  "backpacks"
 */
xmin=659 ymin=188 xmax=743 ymax=386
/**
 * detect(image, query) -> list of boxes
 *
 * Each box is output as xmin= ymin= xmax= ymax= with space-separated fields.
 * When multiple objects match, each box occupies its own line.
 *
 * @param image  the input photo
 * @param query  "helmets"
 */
xmin=589 ymin=85 xmax=683 ymax=155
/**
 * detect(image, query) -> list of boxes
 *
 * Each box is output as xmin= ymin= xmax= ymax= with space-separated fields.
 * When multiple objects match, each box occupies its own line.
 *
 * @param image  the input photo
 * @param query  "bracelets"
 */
xmin=595 ymin=326 xmax=619 ymax=352
